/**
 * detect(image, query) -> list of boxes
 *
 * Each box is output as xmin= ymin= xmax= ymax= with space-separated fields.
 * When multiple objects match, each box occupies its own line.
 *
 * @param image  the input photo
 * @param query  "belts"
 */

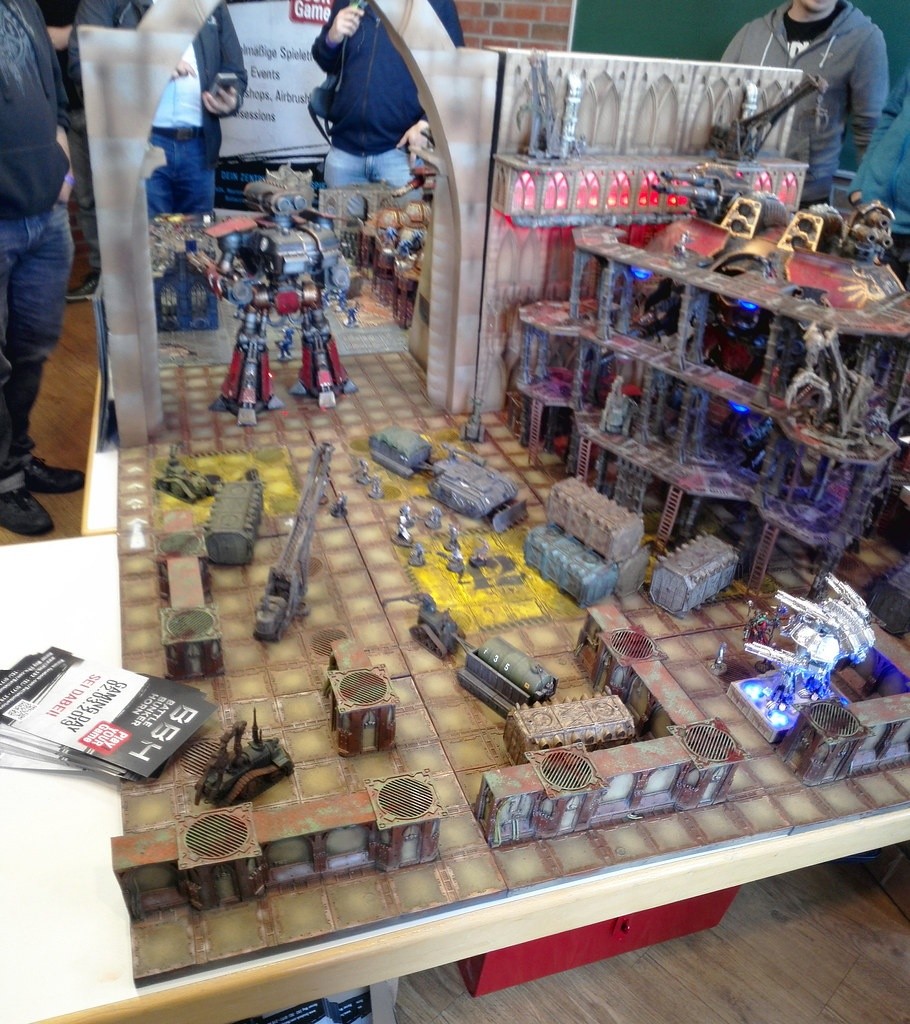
xmin=154 ymin=126 xmax=205 ymax=141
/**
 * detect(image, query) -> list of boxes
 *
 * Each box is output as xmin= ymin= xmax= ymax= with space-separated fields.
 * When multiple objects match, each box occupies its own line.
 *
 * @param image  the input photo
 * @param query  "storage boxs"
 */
xmin=456 ymin=884 xmax=741 ymax=997
xmin=265 ymin=978 xmax=400 ymax=1024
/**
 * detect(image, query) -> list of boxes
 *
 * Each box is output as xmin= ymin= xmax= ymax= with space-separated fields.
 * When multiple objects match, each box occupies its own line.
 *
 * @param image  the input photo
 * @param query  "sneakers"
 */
xmin=65 ymin=274 xmax=96 ymax=302
xmin=22 ymin=456 xmax=85 ymax=494
xmin=0 ymin=489 xmax=54 ymax=535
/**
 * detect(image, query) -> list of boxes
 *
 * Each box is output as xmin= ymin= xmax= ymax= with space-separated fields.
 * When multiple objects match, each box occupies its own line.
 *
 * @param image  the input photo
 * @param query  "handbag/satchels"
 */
xmin=310 ymin=0 xmax=366 ymax=120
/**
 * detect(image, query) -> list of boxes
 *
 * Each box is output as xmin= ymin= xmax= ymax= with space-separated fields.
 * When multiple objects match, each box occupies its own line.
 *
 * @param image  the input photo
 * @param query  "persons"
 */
xmin=721 ymin=0 xmax=890 ymax=211
xmin=0 ymin=0 xmax=247 ymax=537
xmin=846 ymin=63 xmax=910 ymax=289
xmin=311 ymin=0 xmax=465 ymax=229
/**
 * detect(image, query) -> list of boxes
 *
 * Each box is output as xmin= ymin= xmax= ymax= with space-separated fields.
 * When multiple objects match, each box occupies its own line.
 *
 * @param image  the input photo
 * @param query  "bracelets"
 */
xmin=64 ymin=174 xmax=76 ymax=188
xmin=853 ymin=196 xmax=863 ymax=208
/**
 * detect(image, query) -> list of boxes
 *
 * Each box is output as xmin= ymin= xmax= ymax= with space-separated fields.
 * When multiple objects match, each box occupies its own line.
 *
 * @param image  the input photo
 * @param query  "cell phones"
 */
xmin=211 ymin=72 xmax=238 ymax=98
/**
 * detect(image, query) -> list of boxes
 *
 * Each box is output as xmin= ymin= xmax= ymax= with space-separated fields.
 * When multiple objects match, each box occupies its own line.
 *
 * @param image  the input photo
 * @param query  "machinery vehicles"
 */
xmin=706 ymin=72 xmax=828 ymax=160
xmin=194 ymin=707 xmax=296 ymax=809
xmin=153 ymin=442 xmax=211 ymax=503
xmin=251 ymin=441 xmax=334 ymax=640
xmin=429 ymin=441 xmax=528 ymax=534
xmin=381 ymin=592 xmax=466 ymax=660
